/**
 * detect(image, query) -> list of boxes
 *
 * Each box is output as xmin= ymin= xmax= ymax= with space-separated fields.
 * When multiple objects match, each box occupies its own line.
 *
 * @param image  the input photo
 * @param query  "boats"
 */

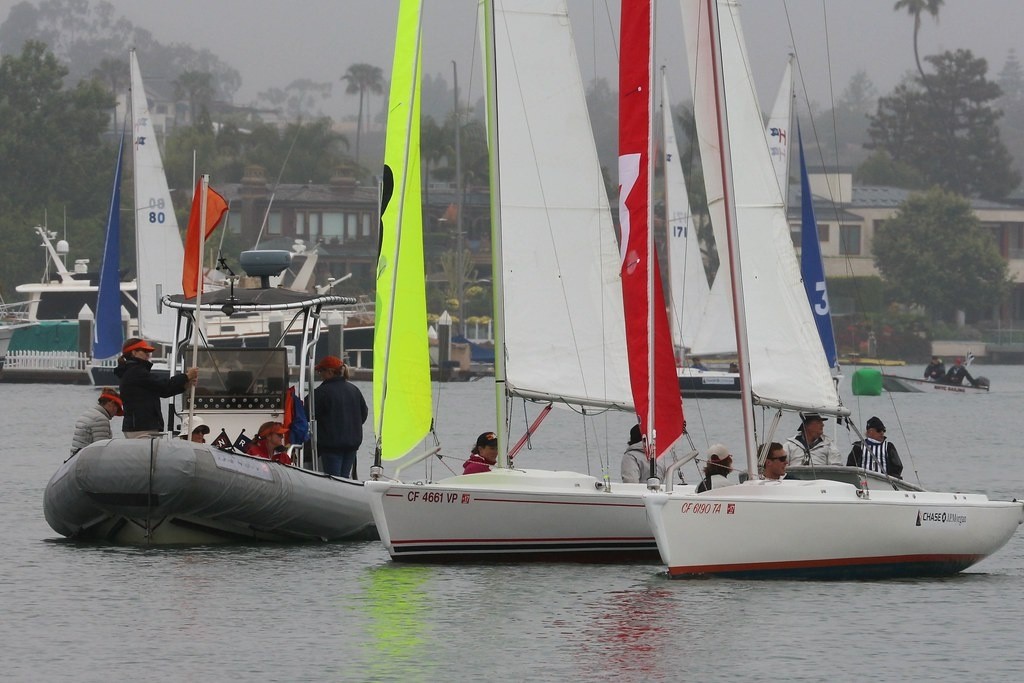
xmin=42 ymin=154 xmax=381 ymax=551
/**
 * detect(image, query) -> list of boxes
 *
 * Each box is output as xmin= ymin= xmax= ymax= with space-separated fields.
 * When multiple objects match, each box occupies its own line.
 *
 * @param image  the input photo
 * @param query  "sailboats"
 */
xmin=618 ymin=1 xmax=1023 ymax=582
xmin=364 ymin=0 xmax=699 ymax=564
xmin=0 ymin=40 xmax=491 ymax=388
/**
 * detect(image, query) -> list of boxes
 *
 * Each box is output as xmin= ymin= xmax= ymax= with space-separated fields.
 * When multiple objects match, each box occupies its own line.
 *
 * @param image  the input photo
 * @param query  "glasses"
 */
xmin=874 ymin=428 xmax=887 ymax=433
xmin=193 ymin=428 xmax=206 ymax=435
xmin=317 ymin=368 xmax=328 ymax=375
xmin=275 ymin=433 xmax=284 ymax=439
xmin=766 ymin=455 xmax=788 ymax=462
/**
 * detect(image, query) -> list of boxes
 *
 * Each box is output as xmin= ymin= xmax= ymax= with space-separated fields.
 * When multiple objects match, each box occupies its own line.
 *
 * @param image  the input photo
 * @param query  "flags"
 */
xmin=963 ymin=348 xmax=975 ymax=367
xmin=234 ymin=434 xmax=254 ymax=454
xmin=212 ymin=432 xmax=232 ymax=450
xmin=182 ymin=178 xmax=231 ymax=300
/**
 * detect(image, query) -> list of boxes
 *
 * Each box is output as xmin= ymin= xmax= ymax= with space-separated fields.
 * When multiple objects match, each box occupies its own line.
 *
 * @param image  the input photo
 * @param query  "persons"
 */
xmin=846 ymin=416 xmax=904 ymax=481
xmin=695 ymin=444 xmax=749 ymax=493
xmin=462 ymin=432 xmax=498 ymax=475
xmin=691 ymin=355 xmax=710 ymax=371
xmin=939 ymin=358 xmax=979 ymax=387
xmin=783 ymin=413 xmax=844 ymax=481
xmin=70 ymin=387 xmax=121 ymax=457
xmin=245 ymin=421 xmax=292 ymax=465
xmin=178 ymin=415 xmax=210 ymax=444
xmin=924 ymin=355 xmax=946 ymax=382
xmin=758 ymin=442 xmax=788 ymax=481
xmin=729 ymin=362 xmax=740 ymax=372
xmin=113 ymin=338 xmax=200 ymax=439
xmin=621 ymin=423 xmax=666 ymax=484
xmin=304 ymin=355 xmax=369 ymax=478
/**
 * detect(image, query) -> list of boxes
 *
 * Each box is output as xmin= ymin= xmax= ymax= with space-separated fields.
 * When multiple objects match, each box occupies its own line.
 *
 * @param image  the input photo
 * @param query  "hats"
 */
xmin=866 ymin=416 xmax=885 ymax=429
xmin=476 ymin=432 xmax=497 ymax=445
xmin=627 ymin=423 xmax=642 ymax=445
xmin=314 ymin=356 xmax=343 ymax=371
xmin=100 ymin=387 xmax=125 ymax=416
xmin=123 ymin=340 xmax=155 ymax=352
xmin=178 ymin=416 xmax=210 ymax=436
xmin=259 ymin=424 xmax=288 ymax=437
xmin=707 ymin=443 xmax=733 ymax=461
xmin=804 ymin=413 xmax=828 ymax=422
